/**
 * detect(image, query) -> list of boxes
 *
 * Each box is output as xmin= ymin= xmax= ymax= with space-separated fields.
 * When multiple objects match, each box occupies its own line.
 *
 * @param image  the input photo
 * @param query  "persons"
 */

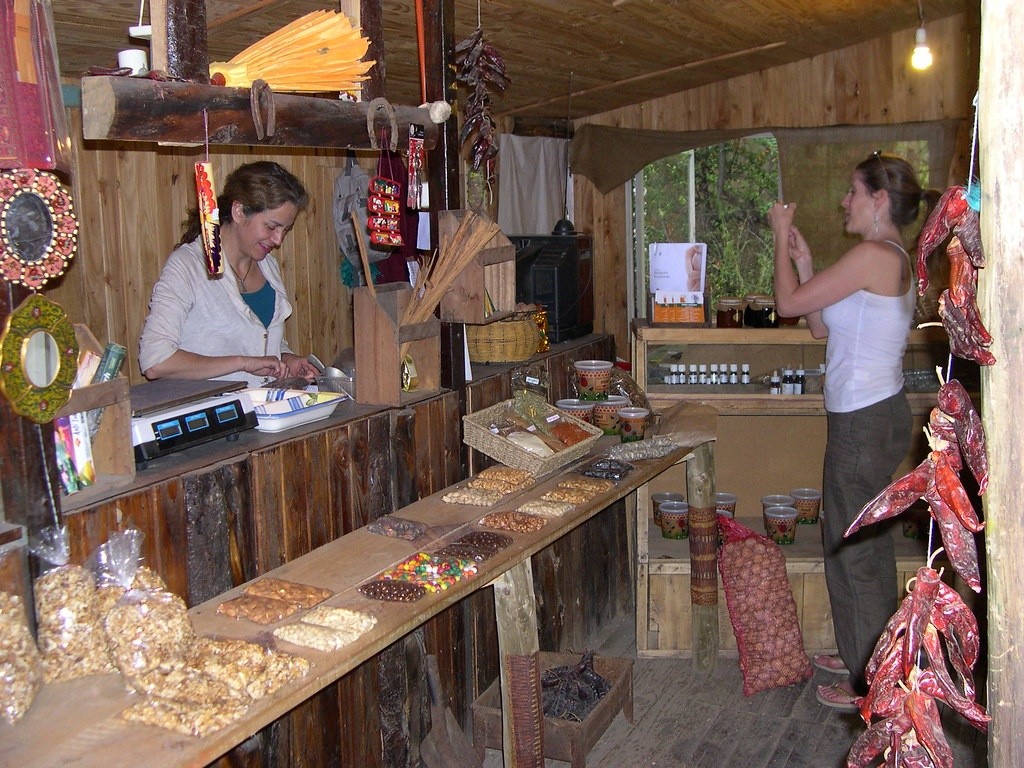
xmin=686 ymin=245 xmax=702 ymax=292
xmin=138 ymin=160 xmax=321 ymax=388
xmin=766 ymin=151 xmax=954 ymax=706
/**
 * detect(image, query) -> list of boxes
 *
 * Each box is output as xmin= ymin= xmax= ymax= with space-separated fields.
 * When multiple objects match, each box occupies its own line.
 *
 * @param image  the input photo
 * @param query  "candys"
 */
xmin=358 ymin=531 xmax=514 ymax=603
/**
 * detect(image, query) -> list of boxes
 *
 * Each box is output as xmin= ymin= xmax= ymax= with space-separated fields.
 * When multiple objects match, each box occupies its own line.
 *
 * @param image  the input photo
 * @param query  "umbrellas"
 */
xmin=209 ymin=10 xmax=377 ymax=93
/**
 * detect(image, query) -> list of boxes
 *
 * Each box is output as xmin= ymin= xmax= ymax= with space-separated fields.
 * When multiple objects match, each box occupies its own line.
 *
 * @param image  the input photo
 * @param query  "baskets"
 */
xmin=463 ymin=400 xmax=603 ymax=479
xmin=465 ymin=320 xmax=539 ymax=363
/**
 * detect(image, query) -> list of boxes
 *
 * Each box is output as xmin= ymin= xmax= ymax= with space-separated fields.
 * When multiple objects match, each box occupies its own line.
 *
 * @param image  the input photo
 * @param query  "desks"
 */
xmin=0 ymin=434 xmax=720 ymax=768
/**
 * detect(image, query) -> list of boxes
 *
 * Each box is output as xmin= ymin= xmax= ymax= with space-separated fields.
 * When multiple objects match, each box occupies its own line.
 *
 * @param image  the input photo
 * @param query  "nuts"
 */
xmin=219 ymin=578 xmax=332 ymax=626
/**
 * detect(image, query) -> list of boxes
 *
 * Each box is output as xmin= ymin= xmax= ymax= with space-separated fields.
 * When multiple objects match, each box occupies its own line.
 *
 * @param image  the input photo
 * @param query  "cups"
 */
xmin=555 ymin=360 xmax=649 ymax=442
xmin=902 ymin=369 xmax=940 ymax=395
xmin=761 ymin=488 xmax=822 ymax=544
xmin=118 ymin=49 xmax=148 ymax=77
xmin=903 ymin=501 xmax=929 ymax=540
xmin=652 ymin=492 xmax=691 ymax=540
xmin=714 ymin=492 xmax=737 ymax=546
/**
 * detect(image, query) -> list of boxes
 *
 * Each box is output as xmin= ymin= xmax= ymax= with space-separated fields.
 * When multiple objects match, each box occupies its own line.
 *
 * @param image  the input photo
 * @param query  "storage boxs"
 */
xmin=351 ymin=278 xmax=441 ymax=408
xmin=468 ymin=650 xmax=636 ymax=768
xmin=53 ymin=323 xmax=137 ymax=508
xmin=437 ymin=208 xmax=516 ymax=325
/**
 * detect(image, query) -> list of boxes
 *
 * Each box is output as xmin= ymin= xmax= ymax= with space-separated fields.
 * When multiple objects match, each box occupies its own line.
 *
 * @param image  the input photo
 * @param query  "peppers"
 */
xmin=841 ymin=183 xmax=995 ymax=768
xmin=453 ymin=26 xmax=513 ymax=185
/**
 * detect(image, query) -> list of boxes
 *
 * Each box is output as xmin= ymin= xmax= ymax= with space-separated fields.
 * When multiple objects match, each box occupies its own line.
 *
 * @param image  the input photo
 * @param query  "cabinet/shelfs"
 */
xmin=630 ymin=313 xmax=984 ymax=665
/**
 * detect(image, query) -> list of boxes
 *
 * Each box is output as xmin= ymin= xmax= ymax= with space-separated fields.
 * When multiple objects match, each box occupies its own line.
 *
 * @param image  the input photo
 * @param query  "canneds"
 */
xmin=715 ymin=294 xmax=801 ymax=329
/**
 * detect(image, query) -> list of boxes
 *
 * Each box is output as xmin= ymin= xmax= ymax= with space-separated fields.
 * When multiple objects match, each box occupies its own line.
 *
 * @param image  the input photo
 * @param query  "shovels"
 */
xmin=306 ymin=354 xmax=355 ymax=402
xmin=418 ymin=655 xmax=482 ymax=768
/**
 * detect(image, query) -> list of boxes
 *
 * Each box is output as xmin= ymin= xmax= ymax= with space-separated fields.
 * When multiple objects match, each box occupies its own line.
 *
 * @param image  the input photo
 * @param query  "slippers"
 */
xmin=811 ymin=652 xmax=851 ymax=674
xmin=817 ymin=683 xmax=859 ymax=708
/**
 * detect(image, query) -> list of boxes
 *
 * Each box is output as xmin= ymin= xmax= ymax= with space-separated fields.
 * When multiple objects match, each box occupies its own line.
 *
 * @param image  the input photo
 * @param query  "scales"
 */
xmin=128 ymin=378 xmax=259 ymax=471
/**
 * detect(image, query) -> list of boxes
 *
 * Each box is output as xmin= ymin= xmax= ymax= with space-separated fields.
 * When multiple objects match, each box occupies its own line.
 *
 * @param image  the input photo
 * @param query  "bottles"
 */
xmin=670 ymin=363 xmax=751 ymax=386
xmin=770 ymin=365 xmax=805 ymax=394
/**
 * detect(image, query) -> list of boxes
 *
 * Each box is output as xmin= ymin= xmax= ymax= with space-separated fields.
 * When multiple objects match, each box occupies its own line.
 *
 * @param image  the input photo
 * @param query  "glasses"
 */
xmin=868 ymin=148 xmax=890 ymax=188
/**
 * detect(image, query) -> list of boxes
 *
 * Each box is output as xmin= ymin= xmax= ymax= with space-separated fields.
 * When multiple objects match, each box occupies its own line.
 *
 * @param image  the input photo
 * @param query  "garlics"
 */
xmin=721 ymin=539 xmax=813 ymax=697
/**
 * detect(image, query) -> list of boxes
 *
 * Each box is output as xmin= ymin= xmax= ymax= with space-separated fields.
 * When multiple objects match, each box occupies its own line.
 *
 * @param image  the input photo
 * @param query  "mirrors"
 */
xmin=0 ymin=167 xmax=80 ymax=427
